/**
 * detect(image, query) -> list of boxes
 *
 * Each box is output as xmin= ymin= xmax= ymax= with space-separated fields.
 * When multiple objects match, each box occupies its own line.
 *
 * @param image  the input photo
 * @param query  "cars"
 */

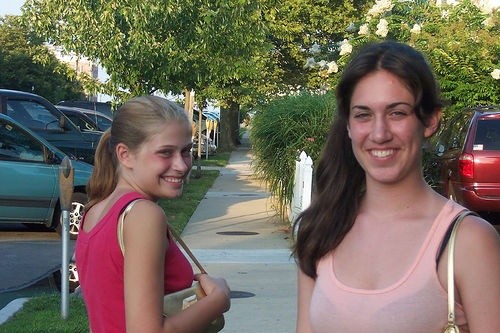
xmin=54 ymin=99 xmax=218 ymax=158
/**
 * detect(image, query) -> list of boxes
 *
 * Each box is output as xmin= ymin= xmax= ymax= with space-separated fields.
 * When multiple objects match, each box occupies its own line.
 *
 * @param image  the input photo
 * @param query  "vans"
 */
xmin=0 ymin=114 xmax=92 ymax=241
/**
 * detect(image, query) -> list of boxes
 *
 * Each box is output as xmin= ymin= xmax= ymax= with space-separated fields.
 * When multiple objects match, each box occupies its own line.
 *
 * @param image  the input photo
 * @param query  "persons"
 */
xmin=73 ymin=95 xmax=232 ymax=333
xmin=291 ymin=40 xmax=500 ymax=333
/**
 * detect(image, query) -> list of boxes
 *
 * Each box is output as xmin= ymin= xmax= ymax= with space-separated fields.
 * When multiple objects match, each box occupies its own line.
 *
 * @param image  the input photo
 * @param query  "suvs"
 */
xmin=0 ymin=88 xmax=106 ymax=166
xmin=426 ymin=108 xmax=500 ymax=220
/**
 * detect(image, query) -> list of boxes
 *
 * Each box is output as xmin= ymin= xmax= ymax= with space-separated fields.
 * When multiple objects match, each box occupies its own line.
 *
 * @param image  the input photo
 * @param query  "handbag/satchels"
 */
xmin=117 ymin=198 xmax=225 ymax=333
